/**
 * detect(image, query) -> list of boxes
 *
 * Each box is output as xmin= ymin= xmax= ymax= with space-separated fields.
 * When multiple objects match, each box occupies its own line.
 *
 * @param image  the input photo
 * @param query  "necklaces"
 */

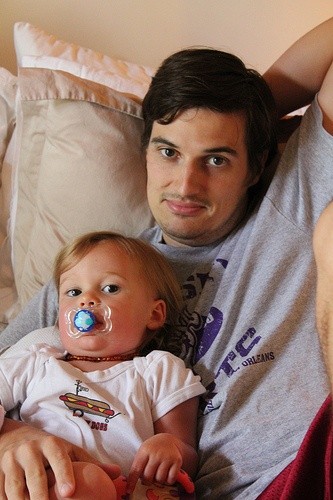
xmin=66 ymin=356 xmax=123 ymax=361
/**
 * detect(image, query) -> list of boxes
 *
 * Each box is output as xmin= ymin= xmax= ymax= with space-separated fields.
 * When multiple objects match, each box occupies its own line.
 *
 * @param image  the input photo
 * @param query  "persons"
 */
xmin=0 ymin=232 xmax=205 ymax=500
xmin=1 ymin=16 xmax=333 ymax=500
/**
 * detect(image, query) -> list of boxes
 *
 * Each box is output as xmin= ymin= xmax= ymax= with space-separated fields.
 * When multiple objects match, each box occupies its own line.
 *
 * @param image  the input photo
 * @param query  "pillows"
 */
xmin=0 ymin=22 xmax=333 ymax=394
